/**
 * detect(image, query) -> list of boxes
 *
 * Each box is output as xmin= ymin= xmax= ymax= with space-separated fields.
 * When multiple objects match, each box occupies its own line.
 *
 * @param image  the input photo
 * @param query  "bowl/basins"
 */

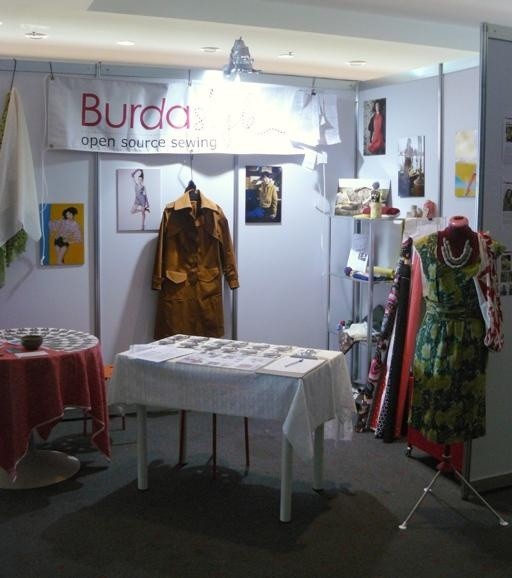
xmin=22 ymin=335 xmax=43 ymax=349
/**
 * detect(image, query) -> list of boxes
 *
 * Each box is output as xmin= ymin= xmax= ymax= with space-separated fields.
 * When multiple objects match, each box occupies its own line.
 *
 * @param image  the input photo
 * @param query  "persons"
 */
xmin=334 ymin=182 xmax=384 ymax=216
xmin=48 ymin=206 xmax=81 ymax=264
xmin=502 ymin=188 xmax=512 ymax=211
xmin=362 ymin=99 xmax=385 ymax=155
xmin=398 ymin=137 xmax=424 ymax=173
xmin=244 ymin=165 xmax=279 ymax=222
xmin=410 ymin=214 xmax=497 ymax=448
xmin=129 ymin=169 xmax=151 ymax=230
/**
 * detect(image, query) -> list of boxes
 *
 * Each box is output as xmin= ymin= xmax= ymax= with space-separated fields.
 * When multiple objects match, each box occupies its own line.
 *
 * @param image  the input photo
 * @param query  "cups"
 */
xmin=414 ymin=180 xmax=424 ymax=197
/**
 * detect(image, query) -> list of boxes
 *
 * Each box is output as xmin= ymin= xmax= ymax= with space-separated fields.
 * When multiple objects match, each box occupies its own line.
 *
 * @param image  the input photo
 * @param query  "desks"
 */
xmin=116 ymin=334 xmax=342 ymax=524
xmin=0 ymin=328 xmax=100 ymax=490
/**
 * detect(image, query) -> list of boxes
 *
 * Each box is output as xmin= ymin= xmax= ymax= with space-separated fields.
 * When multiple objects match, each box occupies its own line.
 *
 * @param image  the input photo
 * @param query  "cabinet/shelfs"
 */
xmin=330 ymin=183 xmax=405 ymax=410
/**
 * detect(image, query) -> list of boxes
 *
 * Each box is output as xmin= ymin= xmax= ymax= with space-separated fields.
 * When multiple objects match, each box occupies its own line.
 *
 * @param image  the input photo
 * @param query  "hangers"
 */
xmin=185 ymin=182 xmax=202 ymax=201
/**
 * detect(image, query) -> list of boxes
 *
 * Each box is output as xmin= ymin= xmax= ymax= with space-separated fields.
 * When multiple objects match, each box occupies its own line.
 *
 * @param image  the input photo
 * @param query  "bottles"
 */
xmin=370 ymin=182 xmax=381 ymax=219
xmin=422 ymin=199 xmax=434 ymax=220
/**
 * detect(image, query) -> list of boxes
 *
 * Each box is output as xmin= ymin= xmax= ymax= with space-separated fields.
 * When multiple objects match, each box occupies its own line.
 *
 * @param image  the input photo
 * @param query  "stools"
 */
xmin=83 ymin=365 xmax=126 ymax=434
